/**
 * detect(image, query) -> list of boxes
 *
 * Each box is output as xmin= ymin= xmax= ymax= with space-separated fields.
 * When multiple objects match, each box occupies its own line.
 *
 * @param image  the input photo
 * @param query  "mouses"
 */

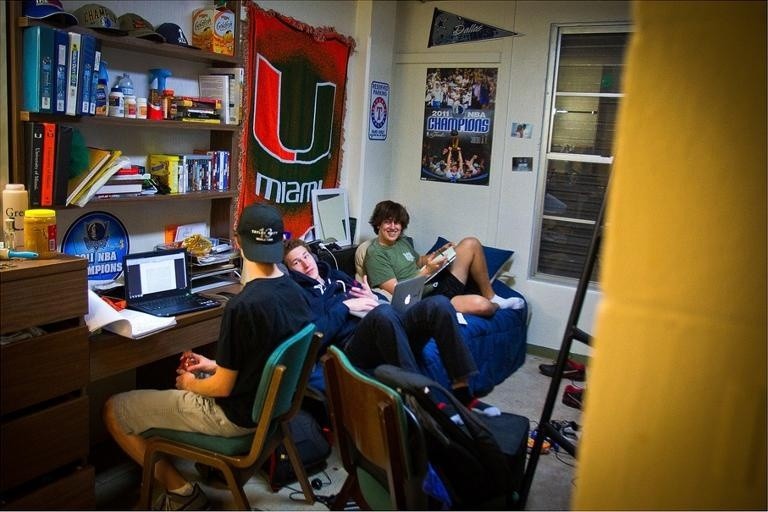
xmin=215 ymin=291 xmax=237 ymax=301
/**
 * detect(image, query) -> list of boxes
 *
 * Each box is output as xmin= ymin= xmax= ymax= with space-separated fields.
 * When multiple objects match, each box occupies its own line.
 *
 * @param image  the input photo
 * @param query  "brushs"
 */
xmin=0 ymin=248 xmax=38 ymax=259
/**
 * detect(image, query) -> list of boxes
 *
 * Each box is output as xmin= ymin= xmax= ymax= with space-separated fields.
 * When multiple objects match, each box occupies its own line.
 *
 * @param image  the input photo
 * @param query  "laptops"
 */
xmin=390 ymin=275 xmax=425 ymax=313
xmin=123 ymin=247 xmax=222 ymax=317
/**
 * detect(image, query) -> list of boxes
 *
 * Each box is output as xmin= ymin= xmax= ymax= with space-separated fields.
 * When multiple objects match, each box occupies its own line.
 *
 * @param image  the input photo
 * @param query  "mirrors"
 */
xmin=310 ymin=186 xmax=351 ymax=247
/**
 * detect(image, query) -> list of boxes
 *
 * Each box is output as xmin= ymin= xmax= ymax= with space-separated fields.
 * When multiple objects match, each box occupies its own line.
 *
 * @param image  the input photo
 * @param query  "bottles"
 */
xmin=2 ymin=182 xmax=30 ymax=252
xmin=98 ymin=61 xmax=147 ymax=120
xmin=160 ymin=89 xmax=178 ymax=120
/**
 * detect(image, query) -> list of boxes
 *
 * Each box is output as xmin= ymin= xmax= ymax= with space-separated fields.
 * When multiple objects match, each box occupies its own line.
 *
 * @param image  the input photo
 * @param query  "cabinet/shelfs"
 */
xmin=8 ymin=18 xmax=239 ymax=284
xmin=1 ymin=249 xmax=98 ymax=510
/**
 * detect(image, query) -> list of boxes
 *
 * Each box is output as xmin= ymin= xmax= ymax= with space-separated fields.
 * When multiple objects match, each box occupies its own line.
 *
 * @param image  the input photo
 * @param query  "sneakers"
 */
xmin=551 ymin=420 xmax=583 ymax=447
xmin=539 ymin=359 xmax=585 ymax=381
xmin=562 ymin=384 xmax=584 ymax=411
xmin=151 ymin=483 xmax=210 ymax=511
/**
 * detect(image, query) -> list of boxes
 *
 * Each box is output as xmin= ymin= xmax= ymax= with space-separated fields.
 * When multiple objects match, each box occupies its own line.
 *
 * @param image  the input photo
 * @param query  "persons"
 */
xmin=363 ymin=200 xmax=525 ymax=317
xmin=425 ymin=68 xmax=495 ymax=109
xmin=103 ymin=202 xmax=311 ymax=511
xmin=421 ymin=138 xmax=487 ymax=179
xmin=282 ymin=239 xmax=501 ymax=425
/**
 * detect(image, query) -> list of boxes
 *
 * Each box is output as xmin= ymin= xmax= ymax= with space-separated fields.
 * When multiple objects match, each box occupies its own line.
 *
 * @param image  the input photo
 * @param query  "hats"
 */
xmin=117 ymin=12 xmax=164 ymax=40
xmin=73 ymin=3 xmax=135 ymax=33
xmin=24 ymin=1 xmax=79 ymax=25
xmin=155 ymin=22 xmax=200 ymax=49
xmin=237 ymin=204 xmax=285 ymax=263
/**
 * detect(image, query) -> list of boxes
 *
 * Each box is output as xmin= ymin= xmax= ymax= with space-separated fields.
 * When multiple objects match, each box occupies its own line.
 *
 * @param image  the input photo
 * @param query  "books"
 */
xmin=26 ymin=122 xmax=129 ymax=206
xmin=199 ymin=67 xmax=244 ymax=125
xmin=83 ymin=289 xmax=177 ymax=340
xmin=23 ymin=24 xmax=103 ymax=116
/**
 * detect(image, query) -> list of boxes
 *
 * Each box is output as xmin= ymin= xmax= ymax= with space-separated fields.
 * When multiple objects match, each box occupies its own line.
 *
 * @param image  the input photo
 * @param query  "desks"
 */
xmin=86 ymin=282 xmax=252 ymax=476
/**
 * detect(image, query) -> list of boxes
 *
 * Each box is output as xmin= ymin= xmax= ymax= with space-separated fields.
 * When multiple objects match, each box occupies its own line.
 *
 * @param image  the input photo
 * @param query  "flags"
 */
xmin=233 ymin=0 xmax=356 ymax=244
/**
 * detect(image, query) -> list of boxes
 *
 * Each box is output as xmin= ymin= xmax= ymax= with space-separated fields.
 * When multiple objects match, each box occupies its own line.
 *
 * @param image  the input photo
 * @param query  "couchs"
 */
xmin=303 ymin=246 xmax=528 ymax=405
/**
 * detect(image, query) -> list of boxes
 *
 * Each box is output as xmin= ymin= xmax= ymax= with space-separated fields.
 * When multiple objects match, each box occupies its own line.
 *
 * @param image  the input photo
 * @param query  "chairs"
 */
xmin=318 ymin=344 xmax=531 ymax=509
xmin=131 ymin=319 xmax=321 ymax=511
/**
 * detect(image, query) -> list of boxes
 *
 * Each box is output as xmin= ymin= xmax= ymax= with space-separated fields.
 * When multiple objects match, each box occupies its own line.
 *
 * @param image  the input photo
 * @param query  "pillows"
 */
xmin=477 ymin=244 xmax=515 ymax=288
xmin=424 ymin=236 xmax=452 ymax=255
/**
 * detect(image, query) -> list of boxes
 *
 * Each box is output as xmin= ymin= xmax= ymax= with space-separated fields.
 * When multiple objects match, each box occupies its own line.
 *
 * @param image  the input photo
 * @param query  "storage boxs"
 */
xmin=191 ymin=5 xmax=235 ymax=58
